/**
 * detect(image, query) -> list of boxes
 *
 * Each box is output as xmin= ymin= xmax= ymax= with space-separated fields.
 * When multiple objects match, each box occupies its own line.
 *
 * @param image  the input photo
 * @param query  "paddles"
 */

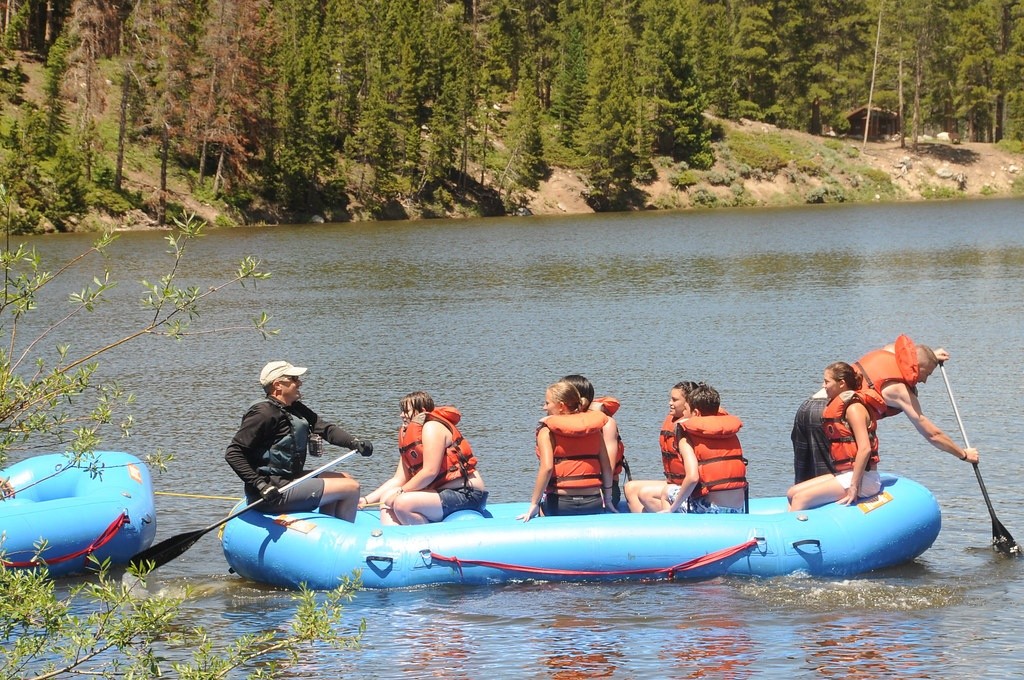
xmin=129 ymin=442 xmax=364 ymax=576
xmin=935 ymin=357 xmax=1024 ymax=560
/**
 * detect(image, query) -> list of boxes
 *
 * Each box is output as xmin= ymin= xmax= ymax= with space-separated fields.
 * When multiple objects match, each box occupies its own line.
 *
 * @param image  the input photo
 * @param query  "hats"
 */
xmin=260 ymin=361 xmax=307 ymax=386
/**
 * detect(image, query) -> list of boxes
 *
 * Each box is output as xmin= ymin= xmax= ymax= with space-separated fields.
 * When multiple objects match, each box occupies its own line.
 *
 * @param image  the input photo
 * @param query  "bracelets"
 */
xmin=959 ymin=450 xmax=967 ymax=461
xmin=602 ymin=485 xmax=612 ymax=489
xmin=364 ymin=497 xmax=368 ymax=504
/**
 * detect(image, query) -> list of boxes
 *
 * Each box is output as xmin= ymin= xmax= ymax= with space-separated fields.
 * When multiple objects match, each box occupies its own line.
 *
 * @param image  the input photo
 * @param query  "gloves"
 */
xmin=255 ymin=480 xmax=280 ymax=503
xmin=353 ymin=440 xmax=373 ymax=456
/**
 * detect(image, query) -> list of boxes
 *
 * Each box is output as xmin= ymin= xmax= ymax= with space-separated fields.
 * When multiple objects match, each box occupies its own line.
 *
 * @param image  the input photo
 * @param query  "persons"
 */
xmin=787 ymin=362 xmax=882 ymax=513
xmin=517 ymin=374 xmax=624 ymax=523
xmin=790 ymin=334 xmax=979 ymax=487
xmin=357 ymin=392 xmax=489 ymax=526
xmin=225 ymin=361 xmax=374 ymax=523
xmin=657 ymin=384 xmax=748 ymax=513
xmin=624 ymin=381 xmax=703 ymax=513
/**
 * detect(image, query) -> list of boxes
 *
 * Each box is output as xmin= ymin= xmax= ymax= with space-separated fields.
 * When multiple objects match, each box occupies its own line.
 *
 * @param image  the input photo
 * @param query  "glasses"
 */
xmin=282 ymin=376 xmax=299 ymax=382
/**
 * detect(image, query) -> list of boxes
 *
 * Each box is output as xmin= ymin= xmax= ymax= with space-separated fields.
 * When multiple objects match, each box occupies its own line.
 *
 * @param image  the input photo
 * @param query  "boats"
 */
xmin=219 ymin=471 xmax=941 ymax=592
xmin=0 ymin=450 xmax=157 ymax=581
